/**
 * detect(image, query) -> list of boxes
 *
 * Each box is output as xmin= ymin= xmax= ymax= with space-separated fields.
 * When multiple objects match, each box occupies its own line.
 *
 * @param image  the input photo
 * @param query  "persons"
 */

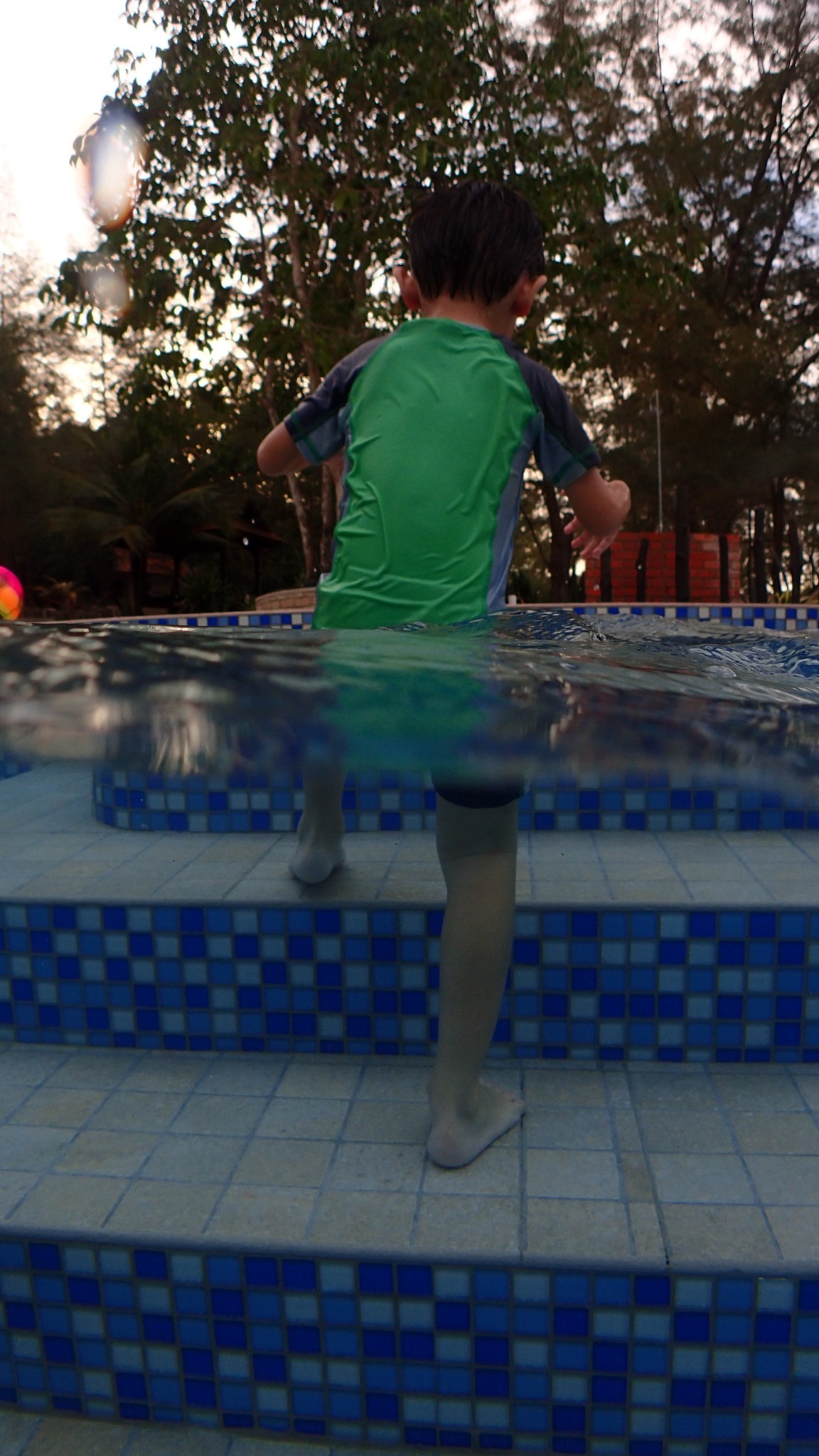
xmin=255 ymin=181 xmax=632 ymax=1175
